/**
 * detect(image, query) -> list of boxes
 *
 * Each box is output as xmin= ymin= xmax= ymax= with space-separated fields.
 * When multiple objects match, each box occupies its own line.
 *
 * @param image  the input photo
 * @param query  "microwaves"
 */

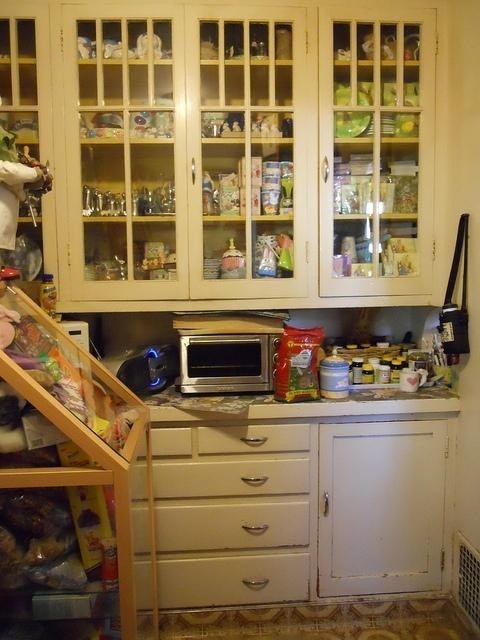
xmin=179 ymin=328 xmax=281 ymax=394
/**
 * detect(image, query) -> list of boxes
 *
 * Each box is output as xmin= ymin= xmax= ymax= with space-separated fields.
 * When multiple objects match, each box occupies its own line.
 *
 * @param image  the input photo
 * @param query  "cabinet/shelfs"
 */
xmin=313 ymin=2 xmax=454 ymax=313
xmin=130 ymin=419 xmax=314 ymax=616
xmin=0 ymin=1 xmax=64 ymax=316
xmin=60 ymin=2 xmax=314 ymax=315
xmin=315 ymin=407 xmax=458 ymax=609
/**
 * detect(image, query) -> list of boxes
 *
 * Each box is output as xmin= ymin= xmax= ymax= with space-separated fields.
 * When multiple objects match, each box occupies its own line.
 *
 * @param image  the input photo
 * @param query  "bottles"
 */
xmin=82 ymin=183 xmax=177 ymax=217
xmin=334 ymin=225 xmax=382 ymax=279
xmin=320 ymin=335 xmax=431 ymax=400
xmin=38 ymin=272 xmax=58 ymax=324
xmin=100 ymin=539 xmax=120 ymax=592
xmin=203 ymin=232 xmax=283 ymax=280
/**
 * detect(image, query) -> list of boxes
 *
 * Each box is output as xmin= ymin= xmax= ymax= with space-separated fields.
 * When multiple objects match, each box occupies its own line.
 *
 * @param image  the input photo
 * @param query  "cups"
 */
xmin=434 ymin=365 xmax=454 ymax=389
xmin=203 ymin=154 xmax=293 ymax=216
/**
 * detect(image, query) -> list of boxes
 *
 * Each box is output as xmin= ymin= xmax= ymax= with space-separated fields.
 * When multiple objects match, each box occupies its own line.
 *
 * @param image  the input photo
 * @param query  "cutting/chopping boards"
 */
xmin=173 ymin=317 xmax=284 ymax=333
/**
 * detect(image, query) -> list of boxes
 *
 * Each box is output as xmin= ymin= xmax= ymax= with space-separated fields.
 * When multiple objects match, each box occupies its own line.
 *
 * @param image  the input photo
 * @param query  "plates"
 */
xmin=0 ymin=235 xmax=44 ymax=281
xmin=334 ymin=79 xmax=419 ymax=139
xmin=11 ymin=118 xmax=38 ymax=139
xmin=88 ymin=104 xmax=176 ymax=139
